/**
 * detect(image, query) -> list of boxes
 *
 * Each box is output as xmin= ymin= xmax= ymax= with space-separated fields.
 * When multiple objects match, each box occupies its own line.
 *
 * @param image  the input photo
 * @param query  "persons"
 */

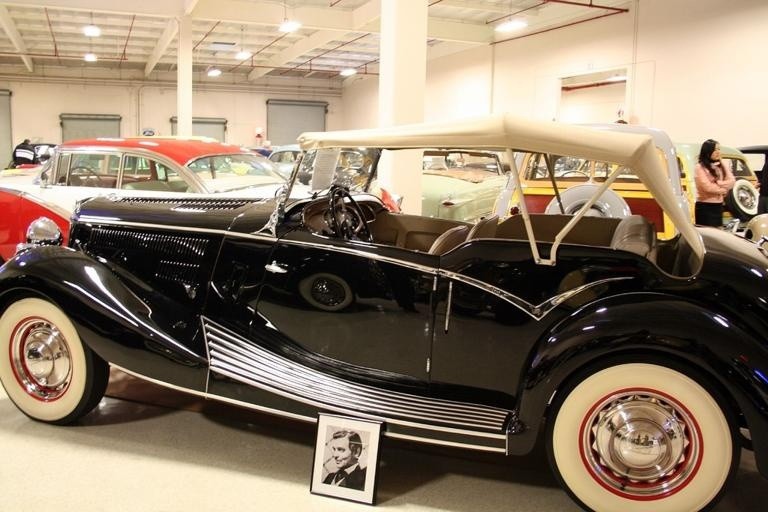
xmin=755 ymin=160 xmax=768 ymax=215
xmin=323 ymin=431 xmax=367 ymax=492
xmin=255 ymin=135 xmax=269 ymax=149
xmin=13 ymin=139 xmax=39 ymax=166
xmin=694 ymin=139 xmax=736 ymax=227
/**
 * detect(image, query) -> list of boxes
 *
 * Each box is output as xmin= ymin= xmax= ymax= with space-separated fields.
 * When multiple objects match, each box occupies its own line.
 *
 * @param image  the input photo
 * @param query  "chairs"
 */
xmin=168 ymin=180 xmax=187 ymax=191
xmin=122 ymin=180 xmax=168 ymax=192
xmin=465 ymin=215 xmax=499 ymax=241
xmin=427 ymin=214 xmax=469 ymax=256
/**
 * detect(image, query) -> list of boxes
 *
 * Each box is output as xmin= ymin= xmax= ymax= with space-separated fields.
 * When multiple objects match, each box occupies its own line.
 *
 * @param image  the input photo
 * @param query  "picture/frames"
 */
xmin=308 ymin=413 xmax=382 ymax=506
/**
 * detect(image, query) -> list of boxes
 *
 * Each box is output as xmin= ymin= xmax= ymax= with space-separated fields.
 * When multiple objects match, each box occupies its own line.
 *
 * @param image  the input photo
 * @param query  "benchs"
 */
xmin=610 ymin=215 xmax=652 ymax=258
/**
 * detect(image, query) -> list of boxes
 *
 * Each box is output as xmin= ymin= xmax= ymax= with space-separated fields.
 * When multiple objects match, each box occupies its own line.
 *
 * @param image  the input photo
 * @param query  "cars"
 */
xmin=244 ymin=245 xmax=608 ymax=327
xmin=0 ymin=113 xmax=767 ymax=288
xmin=0 ymin=109 xmax=767 ymax=512
